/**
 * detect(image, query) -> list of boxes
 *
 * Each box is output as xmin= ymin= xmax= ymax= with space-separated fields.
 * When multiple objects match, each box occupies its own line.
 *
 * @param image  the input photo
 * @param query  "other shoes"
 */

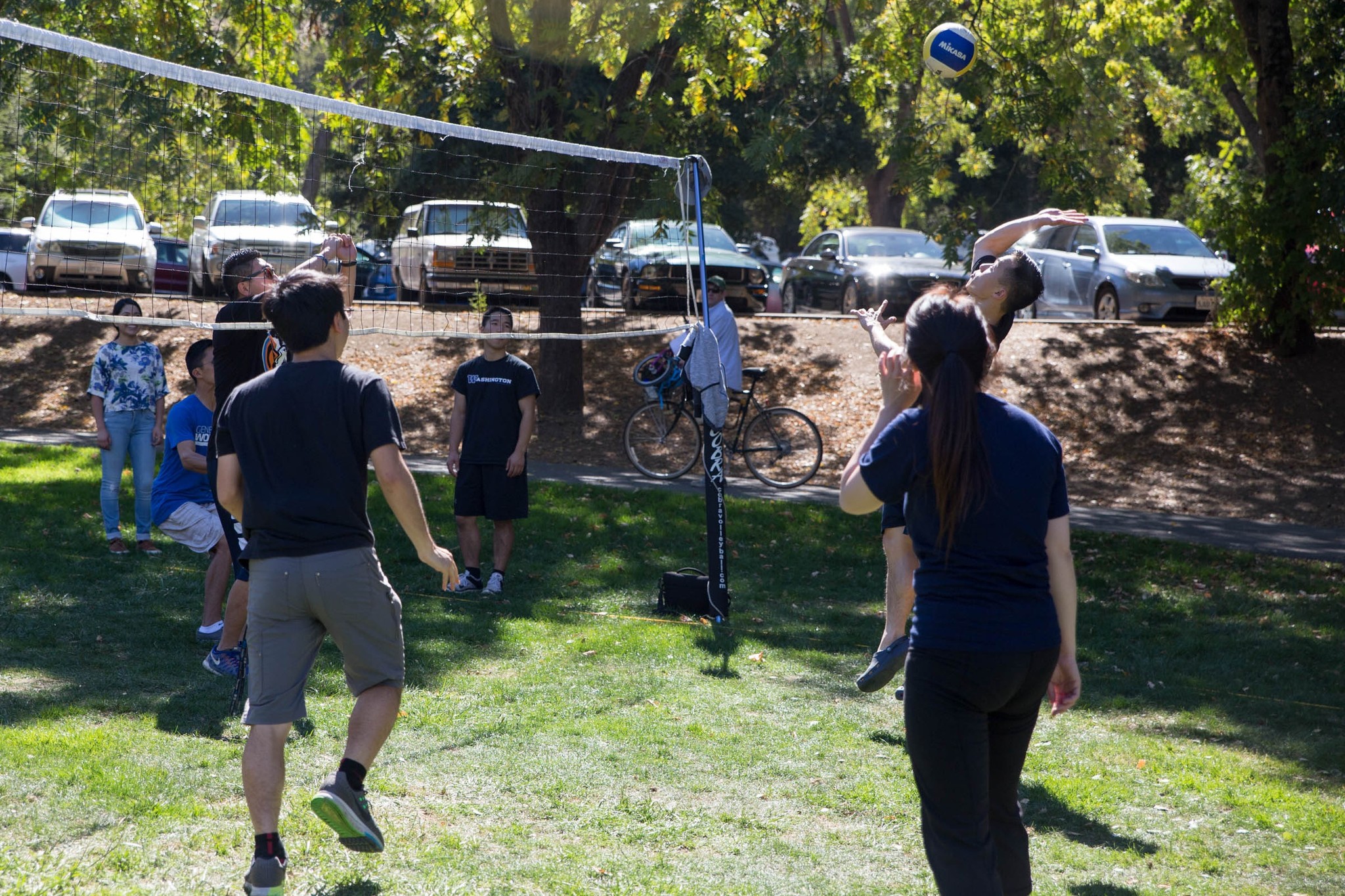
xmin=108 ymin=537 xmax=130 ymax=554
xmin=137 ymin=541 xmax=162 ymax=554
xmin=197 ymin=626 xmax=224 ymax=642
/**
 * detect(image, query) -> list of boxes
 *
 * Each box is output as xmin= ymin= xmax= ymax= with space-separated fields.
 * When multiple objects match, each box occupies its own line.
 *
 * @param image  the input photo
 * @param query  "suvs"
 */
xmin=1003 ymin=216 xmax=1238 ymax=324
xmin=0 ymin=188 xmax=190 ymax=297
xmin=189 ymin=191 xmax=342 ymax=298
xmin=354 ymin=200 xmax=540 ymax=305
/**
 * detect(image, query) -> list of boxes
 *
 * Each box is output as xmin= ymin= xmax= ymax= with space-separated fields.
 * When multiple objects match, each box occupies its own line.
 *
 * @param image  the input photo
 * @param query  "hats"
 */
xmin=674 ymin=154 xmax=712 ymax=205
xmin=707 ymin=275 xmax=727 ymax=290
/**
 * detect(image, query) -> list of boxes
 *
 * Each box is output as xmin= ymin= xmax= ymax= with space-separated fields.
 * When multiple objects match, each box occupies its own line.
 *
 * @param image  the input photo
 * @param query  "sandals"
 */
xmin=895 ymin=682 xmax=905 ymax=701
xmin=856 ymin=635 xmax=910 ymax=693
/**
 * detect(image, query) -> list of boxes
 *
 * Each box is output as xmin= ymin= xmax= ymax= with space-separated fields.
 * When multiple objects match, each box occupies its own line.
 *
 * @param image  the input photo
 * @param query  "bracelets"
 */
xmin=340 ymin=259 xmax=357 ymax=267
xmin=313 ymin=254 xmax=329 ymax=268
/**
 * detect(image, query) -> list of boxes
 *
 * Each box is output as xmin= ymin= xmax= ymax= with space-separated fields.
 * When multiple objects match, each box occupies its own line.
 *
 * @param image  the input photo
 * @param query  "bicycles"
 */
xmin=624 ymin=348 xmax=823 ymax=488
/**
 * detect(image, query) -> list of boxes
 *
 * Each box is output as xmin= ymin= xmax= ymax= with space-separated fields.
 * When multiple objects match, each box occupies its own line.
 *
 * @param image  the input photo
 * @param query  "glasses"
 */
xmin=338 ymin=308 xmax=354 ymax=319
xmin=241 ymin=267 xmax=278 ymax=283
xmin=708 ymin=286 xmax=722 ymax=293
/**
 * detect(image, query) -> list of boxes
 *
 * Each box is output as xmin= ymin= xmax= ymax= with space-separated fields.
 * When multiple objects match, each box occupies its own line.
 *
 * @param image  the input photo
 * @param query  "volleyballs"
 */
xmin=923 ymin=23 xmax=978 ymax=79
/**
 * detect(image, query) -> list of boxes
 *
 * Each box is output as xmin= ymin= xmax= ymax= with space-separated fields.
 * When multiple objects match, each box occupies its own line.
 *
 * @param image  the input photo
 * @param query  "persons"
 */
xmin=837 ymin=208 xmax=1090 ymax=896
xmin=214 ymin=270 xmax=457 ymax=896
xmin=445 ymin=306 xmax=543 ymax=596
xmin=681 ymin=276 xmax=742 ymax=486
xmin=86 ymin=233 xmax=361 ymax=680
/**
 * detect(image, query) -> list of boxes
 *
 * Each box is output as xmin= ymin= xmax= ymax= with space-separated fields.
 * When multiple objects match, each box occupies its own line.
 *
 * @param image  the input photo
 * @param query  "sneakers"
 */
xmin=244 ymin=838 xmax=288 ymax=895
xmin=311 ymin=770 xmax=384 ymax=853
xmin=481 ymin=572 xmax=504 ymax=596
xmin=202 ymin=644 xmax=249 ymax=678
xmin=446 ymin=570 xmax=484 ymax=593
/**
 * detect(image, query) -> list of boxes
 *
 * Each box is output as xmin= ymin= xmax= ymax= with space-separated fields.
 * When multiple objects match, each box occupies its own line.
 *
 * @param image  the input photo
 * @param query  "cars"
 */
xmin=584 ymin=219 xmax=783 ymax=314
xmin=778 ymin=226 xmax=972 ymax=320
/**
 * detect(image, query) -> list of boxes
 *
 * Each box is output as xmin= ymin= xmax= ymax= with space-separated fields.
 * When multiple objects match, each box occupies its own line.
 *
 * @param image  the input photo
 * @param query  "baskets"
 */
xmin=633 ymin=353 xmax=683 ymax=399
